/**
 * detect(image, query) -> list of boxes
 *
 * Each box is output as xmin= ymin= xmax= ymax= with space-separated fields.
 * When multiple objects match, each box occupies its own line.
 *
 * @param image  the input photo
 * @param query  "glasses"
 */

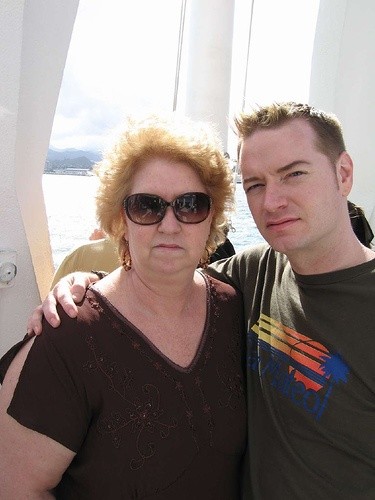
xmin=123 ymin=191 xmax=214 ymax=226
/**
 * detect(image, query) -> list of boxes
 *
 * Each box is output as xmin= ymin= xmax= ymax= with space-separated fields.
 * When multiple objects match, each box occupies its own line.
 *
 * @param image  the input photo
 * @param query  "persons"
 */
xmin=48 ymin=234 xmax=134 ymax=287
xmin=0 ymin=127 xmax=245 ymax=500
xmin=23 ymin=99 xmax=375 ymax=500
